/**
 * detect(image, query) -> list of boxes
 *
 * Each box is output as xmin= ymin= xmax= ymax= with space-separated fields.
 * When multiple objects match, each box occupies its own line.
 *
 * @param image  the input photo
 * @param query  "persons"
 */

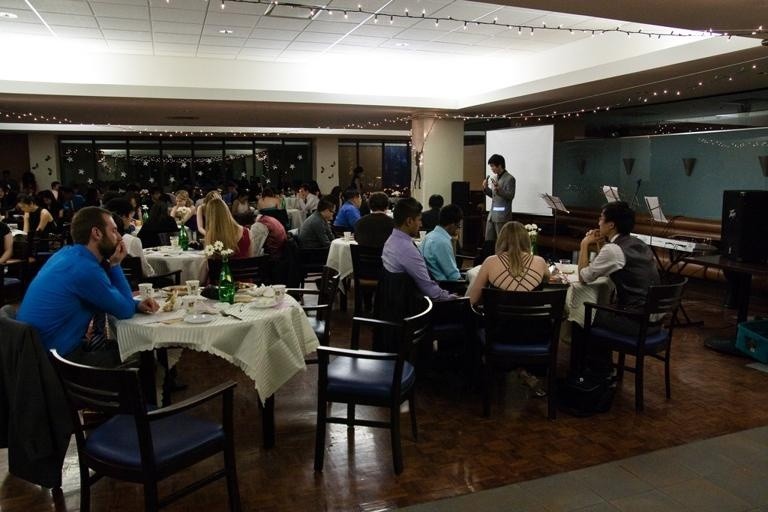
xmin=102 ymin=197 xmax=135 ymax=236
xmin=60 ymin=188 xmax=76 ymax=211
xmin=350 ymin=165 xmax=365 ymax=192
xmin=177 ymin=183 xmax=204 ymax=201
xmin=203 ymin=199 xmax=253 ymax=286
xmin=297 ymin=185 xmax=320 ymax=215
xmin=49 ymin=182 xmax=61 ymax=197
xmin=122 ymin=190 xmax=142 ymax=221
xmin=328 ymin=186 xmax=344 ymax=213
xmin=421 ymin=195 xmax=443 ymax=234
xmin=124 ymin=182 xmax=140 ymax=196
xmin=16 ymin=192 xmax=59 ymax=236
xmin=0 ymin=220 xmax=14 ymax=309
xmin=256 ymin=187 xmax=282 ymax=211
xmin=15 ymin=206 xmax=176 ymax=419
xmin=230 ymin=190 xmax=250 ymax=225
xmin=297 ymin=197 xmax=337 ymax=291
xmin=117 ymin=179 xmax=131 ymax=193
xmin=483 ymin=154 xmax=515 ymax=241
xmin=195 ymin=191 xmax=221 ymax=238
xmin=0 ymin=187 xmax=12 ymax=214
xmin=572 ymin=201 xmax=666 ymax=379
xmin=139 ymin=180 xmax=152 ymax=198
xmin=19 ymin=171 xmax=38 ymax=197
xmin=306 ymin=180 xmax=322 ymax=199
xmin=110 ymin=211 xmax=173 ymax=287
xmin=95 ymin=180 xmax=107 ymax=195
xmin=381 ymin=197 xmax=484 ymax=332
xmin=38 ymin=189 xmax=61 ymax=221
xmin=331 ymin=188 xmax=361 ymax=237
xmin=239 ymin=210 xmax=287 ymax=263
xmin=247 ymin=176 xmax=263 ymax=201
xmin=220 ymin=180 xmax=238 ymax=209
xmin=170 ymin=190 xmax=195 ymax=226
xmin=141 ymin=184 xmax=163 ymax=214
xmin=418 ymin=204 xmax=465 ymax=282
xmin=0 ymin=171 xmax=12 ymax=188
xmin=104 ymin=180 xmax=120 ymax=194
xmin=6 ymin=180 xmax=20 ymax=197
xmin=356 ymin=192 xmax=396 ymax=314
xmin=465 ymin=221 xmax=550 ymax=305
xmin=100 ymin=190 xmax=124 ymax=209
xmin=67 ymin=180 xmax=84 ymax=206
xmin=84 ymin=185 xmax=102 ymax=208
xmin=136 ymin=193 xmax=180 ymax=249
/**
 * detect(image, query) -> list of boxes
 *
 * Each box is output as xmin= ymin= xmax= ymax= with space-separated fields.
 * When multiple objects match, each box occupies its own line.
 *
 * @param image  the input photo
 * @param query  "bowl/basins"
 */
xmin=165 ymin=251 xmax=183 ymax=257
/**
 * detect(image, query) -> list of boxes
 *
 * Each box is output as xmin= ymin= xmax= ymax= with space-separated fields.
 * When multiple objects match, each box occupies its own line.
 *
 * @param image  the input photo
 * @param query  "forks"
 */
xmin=219 ymin=310 xmax=242 ymax=321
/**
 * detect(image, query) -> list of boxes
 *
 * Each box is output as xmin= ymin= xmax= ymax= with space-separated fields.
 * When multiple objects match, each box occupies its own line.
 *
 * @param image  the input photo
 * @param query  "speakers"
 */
xmin=453 ymin=180 xmax=469 ymax=209
xmin=720 ymin=189 xmax=768 ymax=266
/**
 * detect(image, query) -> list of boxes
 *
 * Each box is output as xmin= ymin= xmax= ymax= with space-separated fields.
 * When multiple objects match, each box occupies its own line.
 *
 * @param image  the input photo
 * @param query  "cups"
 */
xmin=419 ymin=231 xmax=426 ymax=240
xmin=170 ymin=236 xmax=179 ymax=250
xmin=560 ymin=259 xmax=570 ymax=277
xmin=272 ymin=285 xmax=285 ymax=303
xmin=138 ymin=283 xmax=154 ymax=300
xmin=344 ymin=232 xmax=351 ymax=242
xmin=186 ymin=281 xmax=199 ymax=297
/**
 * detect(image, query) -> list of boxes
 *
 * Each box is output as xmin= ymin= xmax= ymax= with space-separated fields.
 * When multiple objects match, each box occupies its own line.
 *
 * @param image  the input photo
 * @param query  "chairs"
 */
xmin=258 ymin=209 xmax=293 ymax=237
xmin=582 ymin=277 xmax=688 ymax=415
xmin=380 ymin=266 xmax=471 ymax=394
xmin=121 ymin=257 xmax=183 ymax=292
xmin=314 ymin=295 xmax=433 ymax=476
xmin=49 ymin=348 xmax=242 ymax=512
xmin=208 ymin=253 xmax=272 ymax=288
xmin=264 ymin=264 xmax=341 ymax=406
xmin=478 ymin=287 xmax=567 ymax=421
xmin=289 ymin=227 xmax=330 ymax=296
xmin=350 ymin=243 xmax=385 ymax=317
xmin=1 ymin=305 xmax=77 ymax=512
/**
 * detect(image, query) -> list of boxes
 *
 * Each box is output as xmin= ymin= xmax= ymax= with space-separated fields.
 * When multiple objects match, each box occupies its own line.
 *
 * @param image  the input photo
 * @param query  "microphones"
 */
xmin=484 ymin=175 xmax=492 ymax=181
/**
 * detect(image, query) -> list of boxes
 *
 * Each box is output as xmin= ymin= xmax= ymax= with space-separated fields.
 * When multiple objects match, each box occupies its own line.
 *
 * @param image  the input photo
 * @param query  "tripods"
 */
xmin=552 ymin=209 xmax=557 ymax=261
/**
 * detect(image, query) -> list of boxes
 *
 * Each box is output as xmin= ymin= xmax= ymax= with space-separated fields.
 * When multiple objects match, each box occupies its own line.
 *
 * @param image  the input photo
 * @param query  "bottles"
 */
xmin=218 ymin=254 xmax=234 ymax=304
xmin=531 ymin=241 xmax=538 ymax=255
xmin=281 ymin=198 xmax=286 ymax=209
xmin=142 ymin=208 xmax=149 ymax=224
xmin=179 ymin=221 xmax=188 ymax=251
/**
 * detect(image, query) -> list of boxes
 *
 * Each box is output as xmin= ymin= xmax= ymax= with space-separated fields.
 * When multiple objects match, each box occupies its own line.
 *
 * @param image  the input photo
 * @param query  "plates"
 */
xmin=252 ymin=302 xmax=278 ymax=308
xmin=161 ymin=286 xmax=188 ymax=296
xmin=239 ymin=283 xmax=257 ymax=292
xmin=183 ymin=315 xmax=214 ymax=324
